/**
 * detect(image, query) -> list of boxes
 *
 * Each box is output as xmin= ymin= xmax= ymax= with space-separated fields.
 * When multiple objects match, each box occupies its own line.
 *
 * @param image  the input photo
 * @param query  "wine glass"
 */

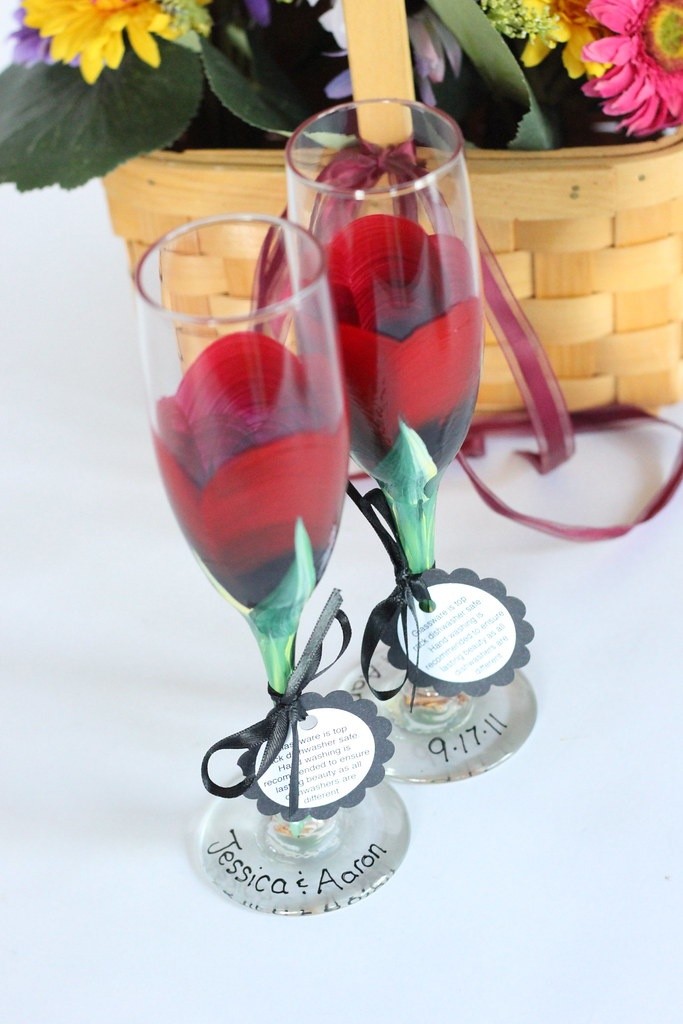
xmin=137 ymin=217 xmax=413 ymax=915
xmin=289 ymin=96 xmax=534 ymax=784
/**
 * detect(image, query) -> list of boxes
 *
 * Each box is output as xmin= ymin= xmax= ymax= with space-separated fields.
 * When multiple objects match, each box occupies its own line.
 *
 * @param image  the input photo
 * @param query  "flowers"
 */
xmin=314 ymin=215 xmax=485 ymax=573
xmin=145 ymin=334 xmax=350 ymax=716
xmin=0 ymin=0 xmax=682 ymax=193
